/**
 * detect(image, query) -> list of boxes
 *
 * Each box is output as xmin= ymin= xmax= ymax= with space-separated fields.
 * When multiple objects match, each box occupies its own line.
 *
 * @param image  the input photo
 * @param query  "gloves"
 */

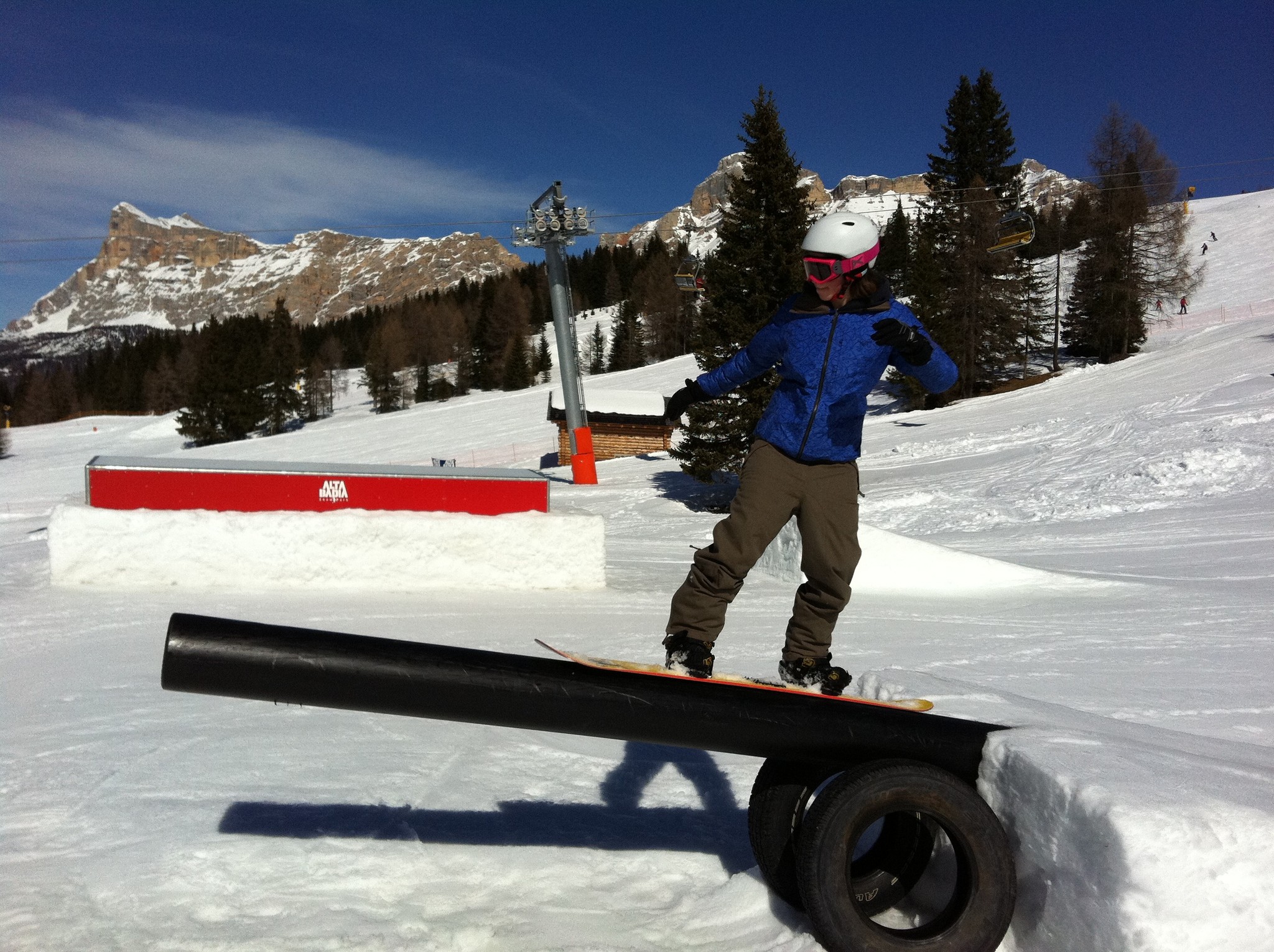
xmin=871 ymin=317 xmax=934 ymax=366
xmin=663 ymin=379 xmax=709 ymax=422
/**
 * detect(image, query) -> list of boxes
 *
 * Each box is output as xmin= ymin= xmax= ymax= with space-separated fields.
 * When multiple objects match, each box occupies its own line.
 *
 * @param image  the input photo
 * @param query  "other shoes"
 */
xmin=662 ymin=631 xmax=714 ymax=678
xmin=779 ymin=654 xmax=851 ymax=695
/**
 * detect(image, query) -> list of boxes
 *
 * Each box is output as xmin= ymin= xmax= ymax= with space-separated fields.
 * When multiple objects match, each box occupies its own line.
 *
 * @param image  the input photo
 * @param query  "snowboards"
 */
xmin=533 ymin=638 xmax=937 ymax=711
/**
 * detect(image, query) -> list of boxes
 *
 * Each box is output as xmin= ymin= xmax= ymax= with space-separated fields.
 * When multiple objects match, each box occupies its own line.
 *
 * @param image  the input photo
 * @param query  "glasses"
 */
xmin=801 ymin=256 xmax=841 ymax=285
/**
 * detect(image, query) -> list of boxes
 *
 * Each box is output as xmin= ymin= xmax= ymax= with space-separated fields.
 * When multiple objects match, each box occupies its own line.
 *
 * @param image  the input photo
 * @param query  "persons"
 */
xmin=1201 ymin=243 xmax=1208 ymax=255
xmin=1180 ymin=296 xmax=1188 ymax=314
xmin=662 ymin=211 xmax=956 ymax=697
xmin=1156 ymin=299 xmax=1162 ymax=310
xmin=1210 ymin=231 xmax=1217 ymax=241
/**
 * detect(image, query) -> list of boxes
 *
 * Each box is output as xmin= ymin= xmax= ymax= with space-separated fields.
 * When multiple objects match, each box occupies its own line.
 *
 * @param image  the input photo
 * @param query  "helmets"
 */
xmin=800 ymin=213 xmax=878 ymax=280
